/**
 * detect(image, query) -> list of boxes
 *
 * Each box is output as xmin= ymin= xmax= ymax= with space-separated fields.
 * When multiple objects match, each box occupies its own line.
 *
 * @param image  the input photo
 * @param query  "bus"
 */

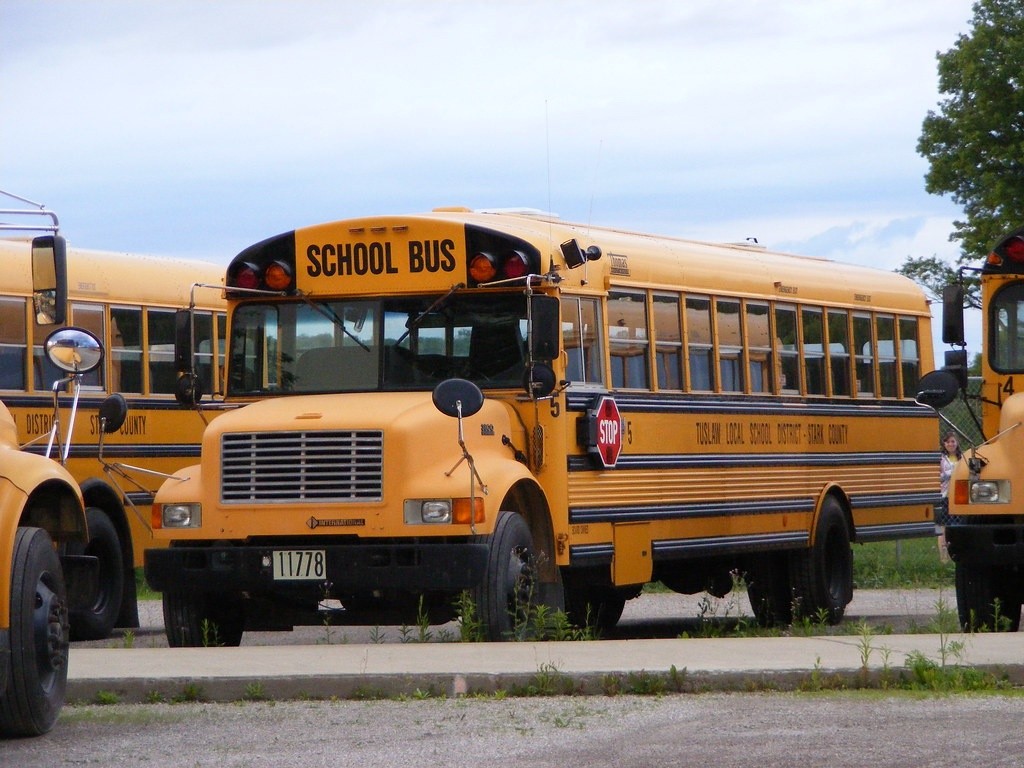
xmin=913 ymin=226 xmax=1024 ymax=631
xmin=95 ymin=204 xmax=946 ymax=649
xmin=0 ymin=185 xmax=105 ymax=737
xmin=0 ymin=236 xmax=229 ymax=640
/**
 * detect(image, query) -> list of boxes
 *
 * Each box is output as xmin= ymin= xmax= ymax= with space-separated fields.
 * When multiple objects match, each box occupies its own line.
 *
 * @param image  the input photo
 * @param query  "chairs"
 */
xmin=466 ymin=313 xmax=525 ymax=382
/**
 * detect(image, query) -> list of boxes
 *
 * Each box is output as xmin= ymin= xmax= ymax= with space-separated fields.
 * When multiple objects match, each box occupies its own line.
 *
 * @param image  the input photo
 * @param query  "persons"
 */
xmin=937 ymin=432 xmax=963 ymax=564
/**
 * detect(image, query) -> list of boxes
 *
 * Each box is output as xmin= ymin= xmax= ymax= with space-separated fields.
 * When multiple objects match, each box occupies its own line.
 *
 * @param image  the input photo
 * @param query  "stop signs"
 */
xmin=595 ymin=395 xmax=622 ymax=469
